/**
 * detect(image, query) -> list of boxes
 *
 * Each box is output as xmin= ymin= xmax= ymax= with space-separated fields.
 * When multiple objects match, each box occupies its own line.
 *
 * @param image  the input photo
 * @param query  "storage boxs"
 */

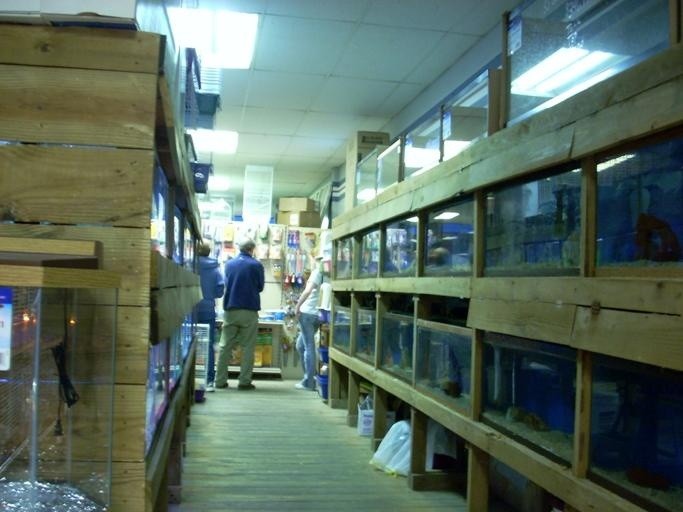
xmin=274 ymin=195 xmax=321 ymax=228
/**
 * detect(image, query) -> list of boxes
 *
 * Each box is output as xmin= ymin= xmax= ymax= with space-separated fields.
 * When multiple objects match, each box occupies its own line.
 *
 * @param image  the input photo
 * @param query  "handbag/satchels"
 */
xmin=357 ymin=403 xmax=397 ymax=436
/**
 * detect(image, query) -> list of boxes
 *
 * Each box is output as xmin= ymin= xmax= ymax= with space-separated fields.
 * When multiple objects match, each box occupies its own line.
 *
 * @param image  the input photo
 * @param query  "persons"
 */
xmin=194 ymin=244 xmax=225 ymax=392
xmin=215 ymin=239 xmax=265 ymax=390
xmin=294 ymin=247 xmax=323 ymax=391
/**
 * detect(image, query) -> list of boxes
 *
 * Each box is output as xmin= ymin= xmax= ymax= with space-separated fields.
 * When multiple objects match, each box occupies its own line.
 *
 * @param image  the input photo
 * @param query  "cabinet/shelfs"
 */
xmin=0 ymin=26 xmax=203 ymax=510
xmin=327 ymin=0 xmax=681 ymax=510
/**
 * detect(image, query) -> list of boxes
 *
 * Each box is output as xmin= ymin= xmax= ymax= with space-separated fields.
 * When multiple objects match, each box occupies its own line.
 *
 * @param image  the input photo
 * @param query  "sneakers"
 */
xmin=204 ymin=382 xmax=214 ymax=391
xmin=238 ymin=385 xmax=256 ymax=390
xmin=294 ymin=381 xmax=315 ymax=391
xmin=218 ymin=383 xmax=228 ymax=388
